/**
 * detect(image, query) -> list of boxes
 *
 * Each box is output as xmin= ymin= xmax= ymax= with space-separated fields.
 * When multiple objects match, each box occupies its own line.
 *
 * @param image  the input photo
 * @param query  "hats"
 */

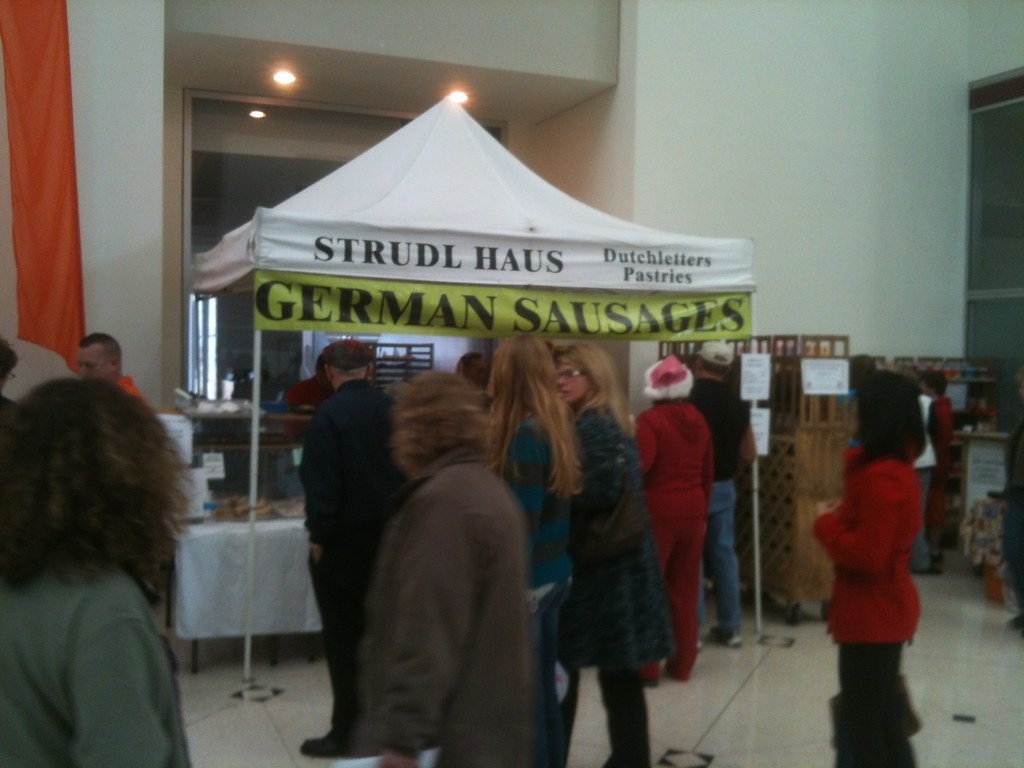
xmin=644 ymin=355 xmax=693 ymax=401
xmin=322 ymin=340 xmax=374 ymax=369
xmin=697 ymin=341 xmax=731 ymax=365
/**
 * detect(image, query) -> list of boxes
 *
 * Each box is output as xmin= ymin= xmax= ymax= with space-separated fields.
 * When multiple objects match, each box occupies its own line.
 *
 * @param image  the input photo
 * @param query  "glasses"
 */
xmin=561 ymin=369 xmax=587 ymax=377
xmin=234 ymin=370 xmax=269 ymax=384
xmin=6 ymin=371 xmax=15 ymax=380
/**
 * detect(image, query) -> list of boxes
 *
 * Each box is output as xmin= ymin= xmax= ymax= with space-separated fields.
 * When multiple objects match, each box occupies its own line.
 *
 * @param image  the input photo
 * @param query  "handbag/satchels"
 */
xmin=570 ymin=491 xmax=640 ymax=560
xmin=829 ymin=676 xmax=920 ymax=738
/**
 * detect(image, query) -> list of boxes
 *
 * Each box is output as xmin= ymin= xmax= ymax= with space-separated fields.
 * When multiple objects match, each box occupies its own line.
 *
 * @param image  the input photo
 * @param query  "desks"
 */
xmin=169 ymin=521 xmax=321 ymax=674
xmin=946 ymin=429 xmax=1011 ymax=535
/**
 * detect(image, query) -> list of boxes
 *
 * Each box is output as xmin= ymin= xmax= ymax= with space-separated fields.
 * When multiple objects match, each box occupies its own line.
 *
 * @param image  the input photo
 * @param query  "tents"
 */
xmin=191 ymin=93 xmax=754 ymax=683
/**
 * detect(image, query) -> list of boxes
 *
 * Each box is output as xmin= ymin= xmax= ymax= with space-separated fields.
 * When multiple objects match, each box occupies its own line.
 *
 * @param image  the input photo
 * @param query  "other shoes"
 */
xmin=665 ymin=664 xmax=684 ymax=679
xmin=1010 ymin=613 xmax=1024 ymax=627
xmin=639 ymin=677 xmax=658 ymax=686
xmin=301 ymin=730 xmax=352 ymax=757
xmin=710 ymin=626 xmax=742 ymax=647
xmin=931 ymin=560 xmax=942 ymax=570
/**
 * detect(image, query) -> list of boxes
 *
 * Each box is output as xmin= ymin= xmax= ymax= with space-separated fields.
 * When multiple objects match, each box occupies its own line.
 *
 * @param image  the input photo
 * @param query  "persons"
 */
xmin=560 ymin=345 xmax=676 ymax=768
xmin=850 ymin=354 xmax=952 ymax=573
xmin=371 ymin=369 xmax=533 ymax=768
xmin=299 ymin=338 xmax=406 ymax=754
xmin=457 ymin=351 xmax=487 ymax=380
xmin=636 ymin=354 xmax=713 ymax=688
xmin=482 ymin=335 xmax=582 ymax=768
xmin=1 ymin=332 xmax=191 ymax=768
xmin=285 ymin=355 xmax=336 ymax=438
xmin=813 ymin=390 xmax=925 ymax=768
xmin=998 ymin=365 xmax=1024 ymax=631
xmin=684 ymin=340 xmax=757 ymax=648
